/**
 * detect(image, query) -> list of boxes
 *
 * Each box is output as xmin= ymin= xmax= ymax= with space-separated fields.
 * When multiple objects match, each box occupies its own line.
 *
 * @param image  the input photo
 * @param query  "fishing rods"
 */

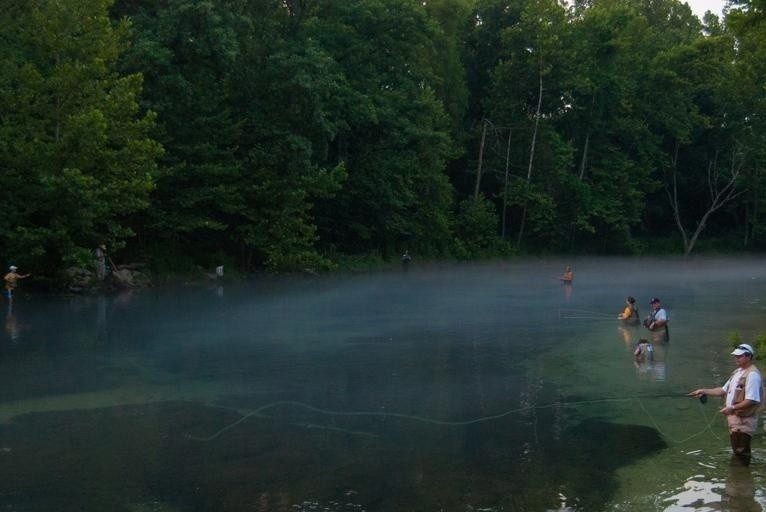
xmin=552 ymin=392 xmax=708 ymax=405
xmin=559 ymin=309 xmax=647 ymax=320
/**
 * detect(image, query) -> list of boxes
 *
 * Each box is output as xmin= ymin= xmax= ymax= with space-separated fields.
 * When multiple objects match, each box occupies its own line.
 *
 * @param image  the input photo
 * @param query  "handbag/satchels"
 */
xmin=644 ymin=316 xmax=657 ymax=330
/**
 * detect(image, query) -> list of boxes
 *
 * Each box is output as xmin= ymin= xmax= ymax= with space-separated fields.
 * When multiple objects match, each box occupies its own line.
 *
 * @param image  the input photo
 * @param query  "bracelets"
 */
xmin=732 ymin=404 xmax=736 ymax=410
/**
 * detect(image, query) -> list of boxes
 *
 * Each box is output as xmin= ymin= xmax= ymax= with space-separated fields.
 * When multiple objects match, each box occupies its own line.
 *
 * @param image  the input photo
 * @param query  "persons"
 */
xmin=632 ymin=337 xmax=654 ymax=361
xmin=642 ymin=297 xmax=669 ymax=345
xmin=3 ymin=265 xmax=31 ymax=301
xmin=652 ymin=344 xmax=667 ymax=383
xmin=615 ymin=295 xmax=640 ymax=325
xmin=93 ymin=296 xmax=110 ymax=338
xmin=562 ymin=284 xmax=573 ymax=301
xmin=558 ymin=265 xmax=573 ymax=286
xmin=400 ymin=249 xmax=411 ymax=272
xmin=217 ymin=283 xmax=225 ymax=299
xmin=632 ymin=360 xmax=654 ymax=388
xmin=5 ymin=299 xmax=31 ymax=343
xmin=214 ymin=263 xmax=224 ymax=282
xmin=684 ymin=342 xmax=762 ymax=455
xmin=693 ymin=455 xmax=764 ymax=512
xmin=618 ymin=324 xmax=638 ymax=353
xmin=94 ymin=243 xmax=108 ymax=280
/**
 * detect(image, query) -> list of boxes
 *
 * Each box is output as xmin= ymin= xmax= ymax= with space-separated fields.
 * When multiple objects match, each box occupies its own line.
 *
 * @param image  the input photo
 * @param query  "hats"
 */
xmin=649 ymin=297 xmax=660 ymax=305
xmin=9 ymin=266 xmax=19 ymax=271
xmin=730 ymin=343 xmax=754 ymax=356
